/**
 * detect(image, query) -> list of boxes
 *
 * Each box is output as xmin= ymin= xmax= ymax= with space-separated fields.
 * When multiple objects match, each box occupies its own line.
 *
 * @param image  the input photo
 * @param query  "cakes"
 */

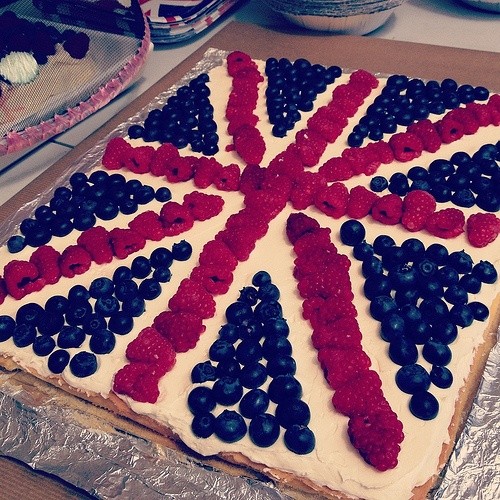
xmin=1 ymin=51 xmax=500 ymax=500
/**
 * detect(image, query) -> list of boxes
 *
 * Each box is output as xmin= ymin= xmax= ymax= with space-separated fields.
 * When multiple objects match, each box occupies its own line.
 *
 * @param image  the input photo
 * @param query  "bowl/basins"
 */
xmin=262 ymin=0 xmax=397 ymax=37
